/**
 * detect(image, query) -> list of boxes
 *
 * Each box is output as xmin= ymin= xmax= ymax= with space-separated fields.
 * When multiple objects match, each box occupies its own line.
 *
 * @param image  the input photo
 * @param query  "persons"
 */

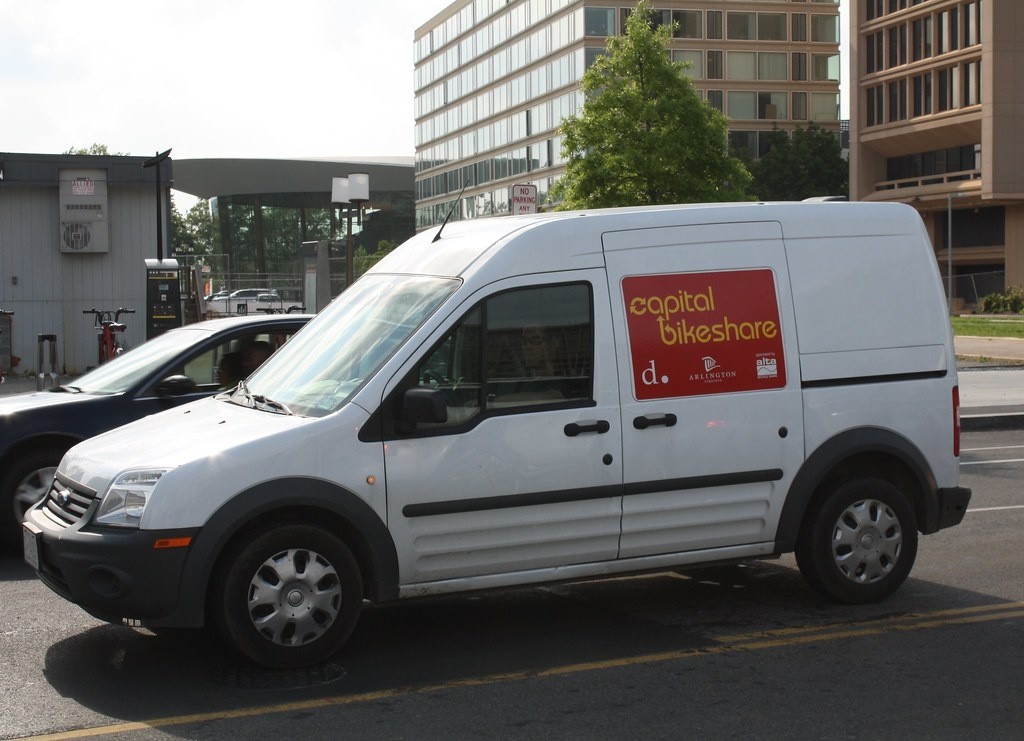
xmin=464 ymin=318 xmax=580 ymax=411
xmin=189 ymin=351 xmax=242 ymax=392
xmin=241 ymin=341 xmax=272 ymax=374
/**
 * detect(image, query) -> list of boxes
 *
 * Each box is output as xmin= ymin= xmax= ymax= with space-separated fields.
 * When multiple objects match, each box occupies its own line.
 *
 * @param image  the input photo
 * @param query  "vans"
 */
xmin=21 ymin=195 xmax=972 ymax=675
xmin=204 ymin=288 xmax=280 ymax=301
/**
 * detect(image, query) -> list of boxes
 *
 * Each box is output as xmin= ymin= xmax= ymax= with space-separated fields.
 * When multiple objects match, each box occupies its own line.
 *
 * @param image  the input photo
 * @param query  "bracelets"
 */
xmin=487 ymin=394 xmax=495 ymax=409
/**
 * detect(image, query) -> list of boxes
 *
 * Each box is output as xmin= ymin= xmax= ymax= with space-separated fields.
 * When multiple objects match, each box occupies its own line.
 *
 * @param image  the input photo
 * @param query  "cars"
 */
xmin=0 ymin=314 xmax=435 ymax=557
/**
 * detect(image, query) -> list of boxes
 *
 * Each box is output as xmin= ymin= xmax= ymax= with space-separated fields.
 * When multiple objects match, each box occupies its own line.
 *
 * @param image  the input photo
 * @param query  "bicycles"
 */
xmin=257 ymin=306 xmax=307 ymax=351
xmin=83 ymin=308 xmax=136 ymax=366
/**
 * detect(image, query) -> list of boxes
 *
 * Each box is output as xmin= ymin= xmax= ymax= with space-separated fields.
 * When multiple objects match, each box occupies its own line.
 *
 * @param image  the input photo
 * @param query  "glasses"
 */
xmin=519 ymin=334 xmax=553 ymax=345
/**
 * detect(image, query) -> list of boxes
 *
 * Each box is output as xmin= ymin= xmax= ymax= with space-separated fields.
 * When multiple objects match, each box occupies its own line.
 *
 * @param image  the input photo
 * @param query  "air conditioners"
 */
xmin=59 ymin=220 xmax=111 ymax=254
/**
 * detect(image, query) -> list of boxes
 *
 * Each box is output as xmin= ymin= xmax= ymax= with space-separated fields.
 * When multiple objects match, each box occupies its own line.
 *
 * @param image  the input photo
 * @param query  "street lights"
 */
xmin=143 ymin=148 xmax=173 ymax=261
xmin=330 ymin=173 xmax=369 ymax=289
